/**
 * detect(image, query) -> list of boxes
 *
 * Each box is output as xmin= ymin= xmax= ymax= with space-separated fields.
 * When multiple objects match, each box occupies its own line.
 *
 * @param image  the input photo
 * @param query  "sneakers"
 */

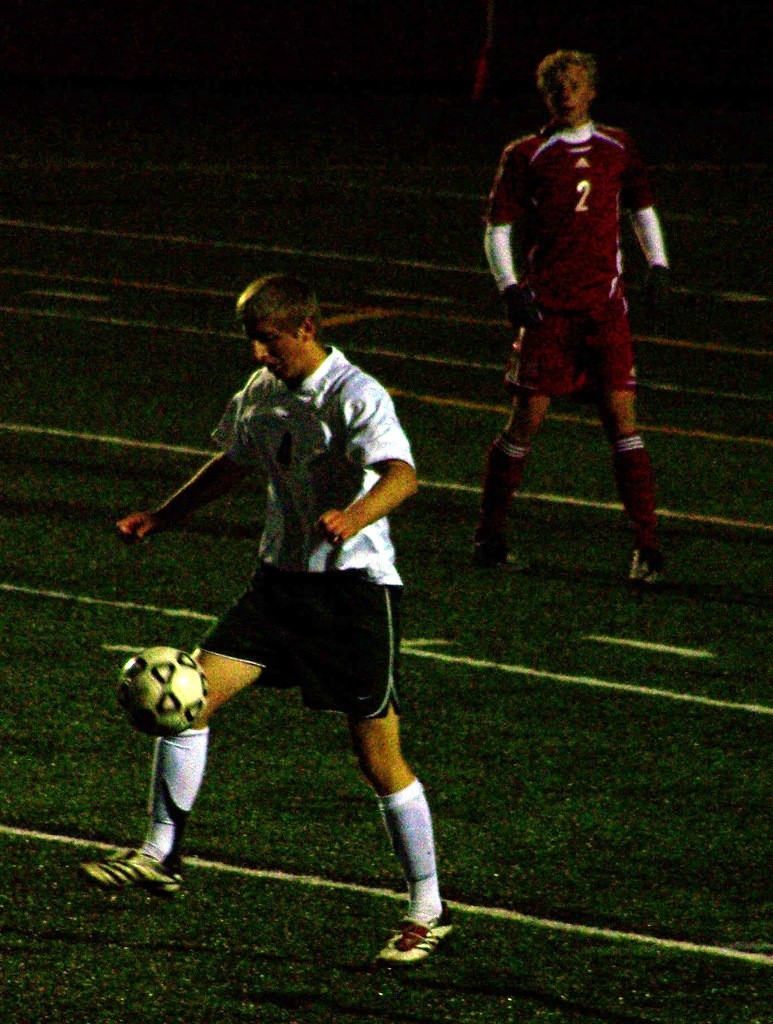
xmin=628 ymin=548 xmax=677 ymax=585
xmin=78 ymin=845 xmax=183 ymax=893
xmin=376 ymin=902 xmax=454 ymax=965
xmin=472 ymin=528 xmax=507 ymax=567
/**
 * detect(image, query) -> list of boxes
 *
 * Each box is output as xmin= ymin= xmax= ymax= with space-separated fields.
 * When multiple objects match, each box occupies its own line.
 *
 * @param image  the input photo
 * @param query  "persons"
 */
xmin=458 ymin=51 xmax=669 ymax=586
xmin=83 ymin=273 xmax=452 ymax=969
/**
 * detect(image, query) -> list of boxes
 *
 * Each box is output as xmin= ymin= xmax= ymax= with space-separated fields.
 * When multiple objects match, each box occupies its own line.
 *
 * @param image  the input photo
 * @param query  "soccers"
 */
xmin=117 ymin=646 xmax=209 ymax=735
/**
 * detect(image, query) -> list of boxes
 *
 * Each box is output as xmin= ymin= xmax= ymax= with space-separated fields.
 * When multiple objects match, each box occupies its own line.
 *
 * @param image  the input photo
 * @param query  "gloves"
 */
xmin=642 ymin=265 xmax=676 ymax=329
xmin=502 ymin=285 xmax=525 ymax=335
